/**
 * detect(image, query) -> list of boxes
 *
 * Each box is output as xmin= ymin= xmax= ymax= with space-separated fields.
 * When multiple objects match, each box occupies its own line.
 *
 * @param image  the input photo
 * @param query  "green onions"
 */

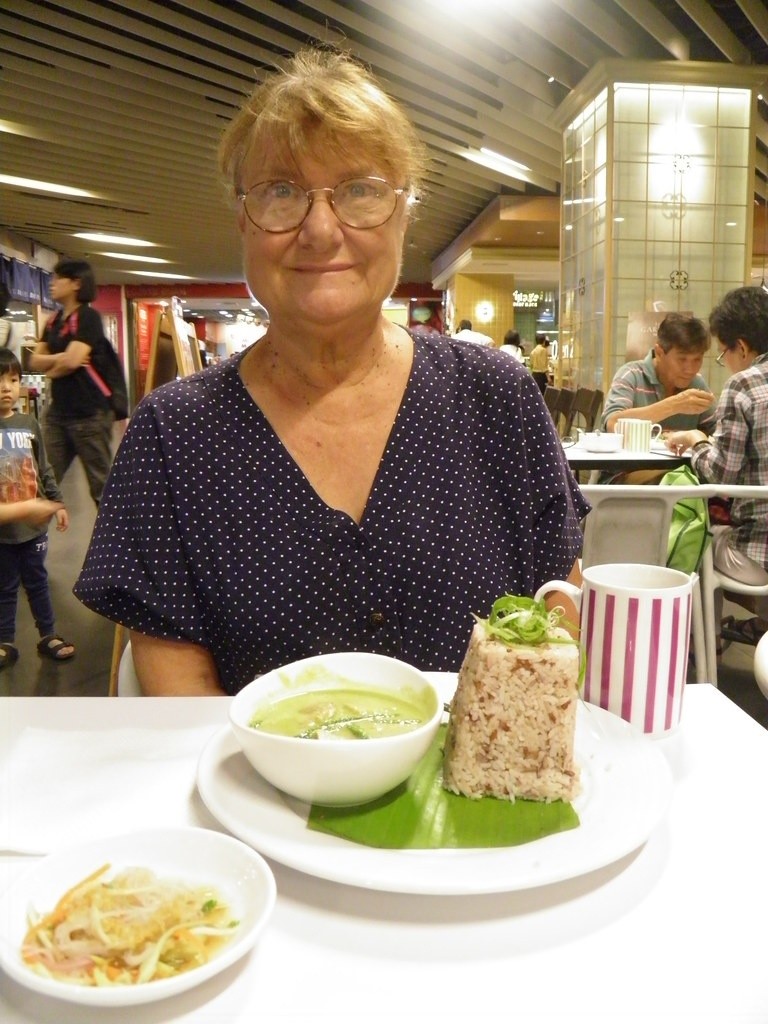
xmin=469 ymin=592 xmax=587 ymax=694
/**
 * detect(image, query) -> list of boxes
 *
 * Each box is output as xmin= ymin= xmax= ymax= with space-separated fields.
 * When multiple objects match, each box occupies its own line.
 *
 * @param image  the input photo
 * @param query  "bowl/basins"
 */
xmin=229 ymin=651 xmax=443 ymax=808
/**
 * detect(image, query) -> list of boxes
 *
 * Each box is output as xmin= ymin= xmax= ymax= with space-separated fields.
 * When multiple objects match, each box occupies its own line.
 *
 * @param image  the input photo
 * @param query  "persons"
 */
xmin=68 ymin=40 xmax=592 ymax=700
xmin=664 ymin=287 xmax=768 ymax=656
xmin=0 ymin=346 xmax=75 ymax=667
xmin=23 ymin=260 xmax=116 ymax=512
xmin=455 ymin=319 xmax=496 ymax=347
xmin=527 ymin=334 xmax=553 ymax=396
xmin=0 ymin=498 xmax=65 ymax=526
xmin=595 ymin=311 xmax=718 ymax=486
xmin=498 ymin=329 xmax=527 ymax=366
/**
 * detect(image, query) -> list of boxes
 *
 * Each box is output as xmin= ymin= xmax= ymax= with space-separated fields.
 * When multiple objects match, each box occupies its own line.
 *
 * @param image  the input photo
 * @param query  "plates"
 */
xmin=0 ymin=824 xmax=277 ymax=1008
xmin=197 ymin=671 xmax=664 ymax=895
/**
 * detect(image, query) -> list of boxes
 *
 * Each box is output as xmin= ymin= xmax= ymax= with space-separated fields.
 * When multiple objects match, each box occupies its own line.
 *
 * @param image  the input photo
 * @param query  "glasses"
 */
xmin=235 ymin=175 xmax=415 ymax=233
xmin=715 ymin=342 xmax=736 ymax=368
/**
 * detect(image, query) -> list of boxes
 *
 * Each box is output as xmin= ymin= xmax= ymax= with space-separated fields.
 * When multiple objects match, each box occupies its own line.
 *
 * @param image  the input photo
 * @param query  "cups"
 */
xmin=615 ymin=418 xmax=661 ymax=451
xmin=533 ymin=563 xmax=693 ymax=740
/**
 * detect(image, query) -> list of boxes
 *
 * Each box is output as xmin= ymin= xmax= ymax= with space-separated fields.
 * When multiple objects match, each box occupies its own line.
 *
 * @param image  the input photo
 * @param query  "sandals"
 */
xmin=0 ymin=644 xmax=18 ymax=669
xmin=37 ymin=634 xmax=75 ymax=659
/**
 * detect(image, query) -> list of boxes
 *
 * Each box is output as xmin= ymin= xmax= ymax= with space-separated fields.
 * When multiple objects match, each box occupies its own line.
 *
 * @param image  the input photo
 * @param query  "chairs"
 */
xmin=543 ymin=386 xmax=603 ymax=485
xmin=575 ymin=484 xmax=768 ymax=698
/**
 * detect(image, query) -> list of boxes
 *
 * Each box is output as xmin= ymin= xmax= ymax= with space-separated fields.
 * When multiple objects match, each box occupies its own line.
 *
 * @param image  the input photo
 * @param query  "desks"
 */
xmin=1 ymin=683 xmax=766 ymax=1024
xmin=562 ymin=433 xmax=692 ymax=469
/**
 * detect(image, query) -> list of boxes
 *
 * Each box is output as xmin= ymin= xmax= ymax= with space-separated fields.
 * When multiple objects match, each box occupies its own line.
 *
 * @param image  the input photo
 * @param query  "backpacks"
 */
xmin=45 ymin=308 xmax=129 ymax=421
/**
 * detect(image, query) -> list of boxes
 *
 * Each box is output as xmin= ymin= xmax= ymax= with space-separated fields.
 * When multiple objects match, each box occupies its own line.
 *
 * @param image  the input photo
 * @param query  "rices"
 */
xmin=440 ymin=622 xmax=581 ymax=804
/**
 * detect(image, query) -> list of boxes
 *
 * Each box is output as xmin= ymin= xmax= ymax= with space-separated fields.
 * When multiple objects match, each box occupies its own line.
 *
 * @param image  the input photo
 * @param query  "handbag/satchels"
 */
xmin=658 ymin=464 xmax=713 ymax=576
xmin=706 ymin=497 xmax=734 ymax=527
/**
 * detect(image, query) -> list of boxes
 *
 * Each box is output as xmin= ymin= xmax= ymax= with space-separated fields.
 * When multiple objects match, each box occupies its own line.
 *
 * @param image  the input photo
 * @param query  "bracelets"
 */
xmin=693 ymin=440 xmax=712 ymax=448
